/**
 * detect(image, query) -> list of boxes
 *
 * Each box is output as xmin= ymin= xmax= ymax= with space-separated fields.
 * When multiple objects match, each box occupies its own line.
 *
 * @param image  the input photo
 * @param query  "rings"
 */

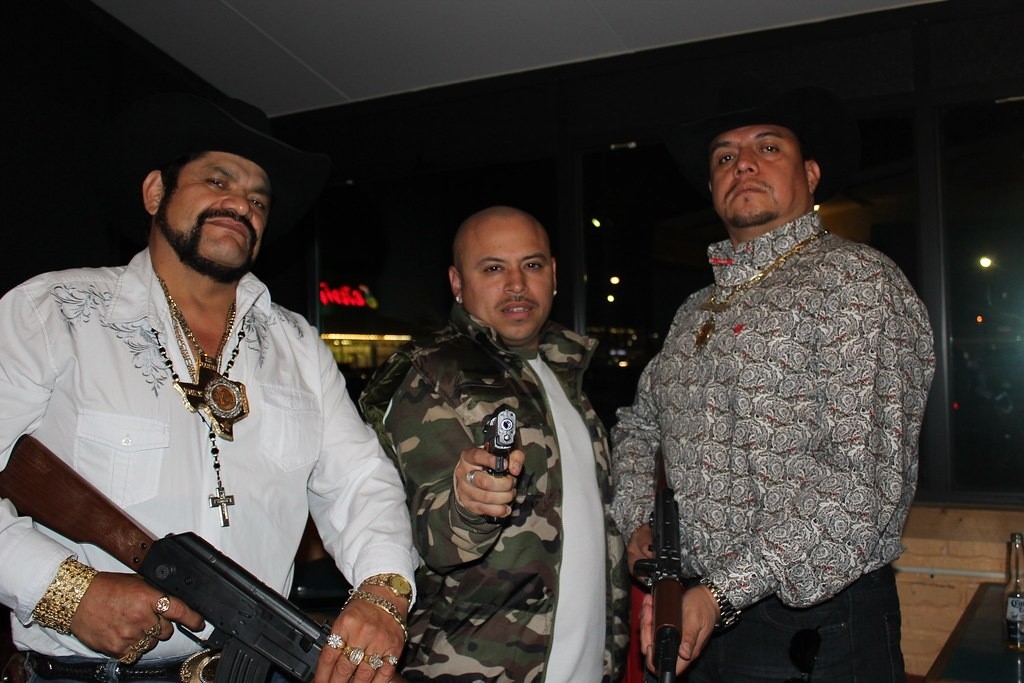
xmin=144 ymin=616 xmax=162 ymax=638
xmin=364 ymin=652 xmax=383 ymax=670
xmin=118 ymin=650 xmax=136 ymax=664
xmin=343 ymin=646 xmax=365 ymax=666
xmin=381 ymin=654 xmax=398 ymax=665
xmin=326 ymin=634 xmax=346 ymax=650
xmin=467 ymin=469 xmax=480 ymax=484
xmin=129 ymin=636 xmax=150 ymax=653
xmin=156 ymin=594 xmax=170 ymax=614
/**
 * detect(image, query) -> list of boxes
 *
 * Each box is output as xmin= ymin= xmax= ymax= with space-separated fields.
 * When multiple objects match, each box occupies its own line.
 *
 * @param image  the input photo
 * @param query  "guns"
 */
xmin=483 ymin=403 xmax=518 ymax=524
xmin=5 ymin=438 xmax=335 ymax=683
xmin=634 ymin=445 xmax=685 ymax=683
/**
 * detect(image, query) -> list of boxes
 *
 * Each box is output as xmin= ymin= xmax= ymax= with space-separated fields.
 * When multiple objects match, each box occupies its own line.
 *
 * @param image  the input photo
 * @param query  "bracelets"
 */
xmin=32 ymin=558 xmax=98 ymax=635
xmin=342 ymin=591 xmax=409 ymax=641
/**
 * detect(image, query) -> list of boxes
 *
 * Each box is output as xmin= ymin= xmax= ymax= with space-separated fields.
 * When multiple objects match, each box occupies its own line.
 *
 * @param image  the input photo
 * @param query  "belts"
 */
xmin=24 ymin=652 xmax=184 ymax=682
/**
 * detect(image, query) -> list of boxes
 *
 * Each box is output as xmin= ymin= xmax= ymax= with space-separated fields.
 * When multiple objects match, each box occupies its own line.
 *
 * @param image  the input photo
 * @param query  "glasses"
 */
xmin=786 ymin=625 xmax=822 ymax=682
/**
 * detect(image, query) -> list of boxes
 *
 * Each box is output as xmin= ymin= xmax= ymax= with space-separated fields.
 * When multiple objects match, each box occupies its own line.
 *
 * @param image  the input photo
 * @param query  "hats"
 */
xmin=658 ymin=78 xmax=847 ymax=198
xmin=120 ymin=93 xmax=331 ymax=238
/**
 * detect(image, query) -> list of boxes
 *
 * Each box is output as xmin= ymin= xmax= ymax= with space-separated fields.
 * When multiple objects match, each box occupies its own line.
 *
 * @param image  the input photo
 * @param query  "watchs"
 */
xmin=699 ymin=577 xmax=740 ymax=634
xmin=358 ymin=573 xmax=413 ymax=608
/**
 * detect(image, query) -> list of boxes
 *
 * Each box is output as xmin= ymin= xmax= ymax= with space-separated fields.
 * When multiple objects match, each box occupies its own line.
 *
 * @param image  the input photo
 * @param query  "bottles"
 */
xmin=1004 ymin=532 xmax=1024 ymax=653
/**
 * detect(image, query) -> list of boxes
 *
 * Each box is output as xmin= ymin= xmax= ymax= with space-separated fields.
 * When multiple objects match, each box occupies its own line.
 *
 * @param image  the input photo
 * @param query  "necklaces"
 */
xmin=692 ymin=228 xmax=830 ymax=351
xmin=144 ymin=314 xmax=246 ymax=529
xmin=156 ymin=275 xmax=250 ymax=442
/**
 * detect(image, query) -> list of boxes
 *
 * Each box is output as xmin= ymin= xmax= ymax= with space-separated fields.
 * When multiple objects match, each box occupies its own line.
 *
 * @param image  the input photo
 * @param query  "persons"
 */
xmin=0 ymin=93 xmax=420 ymax=683
xmin=355 ymin=205 xmax=631 ymax=683
xmin=609 ymin=86 xmax=937 ymax=683
